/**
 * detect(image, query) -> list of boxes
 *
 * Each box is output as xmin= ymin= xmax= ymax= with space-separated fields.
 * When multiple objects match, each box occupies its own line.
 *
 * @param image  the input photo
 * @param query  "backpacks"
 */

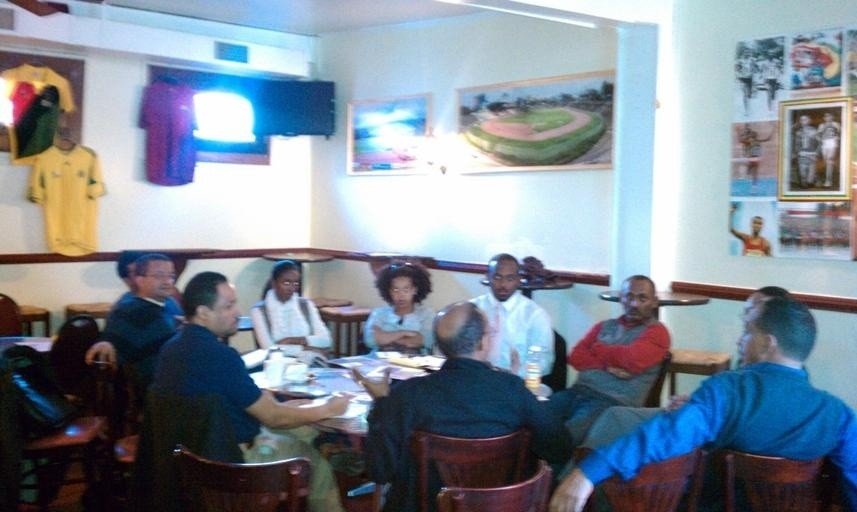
xmin=0 ymin=345 xmax=86 ymax=439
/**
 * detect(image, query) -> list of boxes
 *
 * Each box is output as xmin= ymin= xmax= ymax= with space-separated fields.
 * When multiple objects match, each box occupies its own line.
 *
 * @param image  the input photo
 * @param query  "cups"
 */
xmin=263 ymin=360 xmax=283 ymax=386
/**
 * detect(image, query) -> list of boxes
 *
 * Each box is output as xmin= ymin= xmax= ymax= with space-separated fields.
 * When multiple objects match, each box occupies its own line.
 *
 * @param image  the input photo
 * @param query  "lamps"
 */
xmin=423 ymin=125 xmax=467 ymax=176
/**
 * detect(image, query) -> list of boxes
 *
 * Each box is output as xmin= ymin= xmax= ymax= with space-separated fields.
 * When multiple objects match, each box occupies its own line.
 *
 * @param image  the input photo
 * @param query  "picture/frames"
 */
xmin=777 ymin=96 xmax=853 ymax=202
xmin=454 ymin=68 xmax=617 ymax=174
xmin=345 ymin=92 xmax=433 ymax=176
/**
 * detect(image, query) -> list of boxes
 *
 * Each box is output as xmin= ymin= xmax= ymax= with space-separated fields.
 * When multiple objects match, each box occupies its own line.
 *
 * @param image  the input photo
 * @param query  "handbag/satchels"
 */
xmin=5 ymin=348 xmax=77 ymax=440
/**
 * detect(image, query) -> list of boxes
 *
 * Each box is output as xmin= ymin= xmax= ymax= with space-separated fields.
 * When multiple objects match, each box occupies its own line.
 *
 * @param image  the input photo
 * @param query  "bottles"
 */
xmin=524 ymin=345 xmax=541 ymax=396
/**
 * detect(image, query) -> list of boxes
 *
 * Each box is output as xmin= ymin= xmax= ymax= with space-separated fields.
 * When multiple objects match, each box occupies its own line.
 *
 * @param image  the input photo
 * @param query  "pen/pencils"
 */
xmin=330 ymin=392 xmax=357 ymax=404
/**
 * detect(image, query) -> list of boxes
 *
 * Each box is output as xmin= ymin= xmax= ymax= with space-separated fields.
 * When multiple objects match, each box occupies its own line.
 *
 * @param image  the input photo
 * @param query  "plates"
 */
xmin=285 ymin=374 xmax=318 ymax=383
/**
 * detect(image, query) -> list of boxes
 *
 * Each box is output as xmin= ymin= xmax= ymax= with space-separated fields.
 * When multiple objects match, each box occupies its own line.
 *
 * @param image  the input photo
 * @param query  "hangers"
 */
xmin=23 ymin=52 xmax=50 ymax=71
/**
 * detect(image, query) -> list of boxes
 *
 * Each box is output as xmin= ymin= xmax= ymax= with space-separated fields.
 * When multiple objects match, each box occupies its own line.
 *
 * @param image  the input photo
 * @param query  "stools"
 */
xmin=65 ymin=303 xmax=112 ymax=328
xmin=221 ymin=317 xmax=255 ymax=346
xmin=665 ymin=349 xmax=731 ymax=398
xmin=308 ymin=294 xmax=353 ymax=357
xmin=22 ymin=305 xmax=51 ymax=337
xmin=321 ymin=306 xmax=372 ymax=359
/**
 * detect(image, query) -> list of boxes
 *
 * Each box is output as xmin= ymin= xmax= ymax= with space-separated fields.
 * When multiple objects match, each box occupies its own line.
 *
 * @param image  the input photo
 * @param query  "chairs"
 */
xmin=171 ymin=443 xmax=311 ymax=512
xmin=571 ymin=447 xmax=708 ymax=512
xmin=0 ymin=346 xmax=106 ymax=505
xmin=437 ymin=458 xmax=552 ymax=512
xmin=408 ymin=428 xmax=530 ymax=512
xmin=712 ymin=447 xmax=840 ymax=512
xmin=84 ymin=362 xmax=141 ymax=512
xmin=0 ymin=293 xmax=22 ymax=337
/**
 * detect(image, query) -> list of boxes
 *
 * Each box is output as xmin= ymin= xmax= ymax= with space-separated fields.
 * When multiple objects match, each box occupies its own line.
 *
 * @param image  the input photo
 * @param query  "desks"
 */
xmin=263 ymin=252 xmax=334 ymax=297
xmin=598 ymin=290 xmax=709 ymax=320
xmin=250 ymin=354 xmax=553 ymax=453
xmin=0 ymin=336 xmax=57 ymax=371
xmin=479 ymin=276 xmax=572 ymax=299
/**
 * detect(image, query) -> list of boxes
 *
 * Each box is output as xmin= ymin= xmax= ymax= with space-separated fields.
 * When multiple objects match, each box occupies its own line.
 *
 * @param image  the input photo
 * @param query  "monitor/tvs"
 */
xmin=252 ymin=79 xmax=336 ymax=137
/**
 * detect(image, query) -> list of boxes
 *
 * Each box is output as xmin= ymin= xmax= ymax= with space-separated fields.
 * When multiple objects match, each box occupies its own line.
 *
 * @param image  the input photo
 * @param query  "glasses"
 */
xmin=137 ymin=273 xmax=177 ymax=282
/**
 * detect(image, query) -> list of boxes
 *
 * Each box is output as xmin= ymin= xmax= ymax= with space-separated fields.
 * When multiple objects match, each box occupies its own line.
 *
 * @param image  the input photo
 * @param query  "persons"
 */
xmin=731 ymin=286 xmax=792 ymax=372
xmin=353 ymin=301 xmax=574 ymax=512
xmin=729 ymin=204 xmax=771 ymax=258
xmin=149 ymin=272 xmax=351 ymax=511
xmin=795 ymin=115 xmax=819 ymax=188
xmin=737 ymin=48 xmax=760 ymax=115
xmin=116 ymin=250 xmax=182 ymax=314
xmin=540 ymin=275 xmax=670 ymax=447
xmin=551 ymin=296 xmax=857 ymax=511
xmin=736 ymin=126 xmax=774 ymax=183
xmin=86 ymin=254 xmax=183 ymax=419
xmin=250 ymin=260 xmax=333 ymax=362
xmin=762 ymin=50 xmax=778 ymax=109
xmin=363 ymin=262 xmax=436 ymax=356
xmin=433 ymin=253 xmax=556 ymax=379
xmin=815 ymin=112 xmax=840 ymax=187
